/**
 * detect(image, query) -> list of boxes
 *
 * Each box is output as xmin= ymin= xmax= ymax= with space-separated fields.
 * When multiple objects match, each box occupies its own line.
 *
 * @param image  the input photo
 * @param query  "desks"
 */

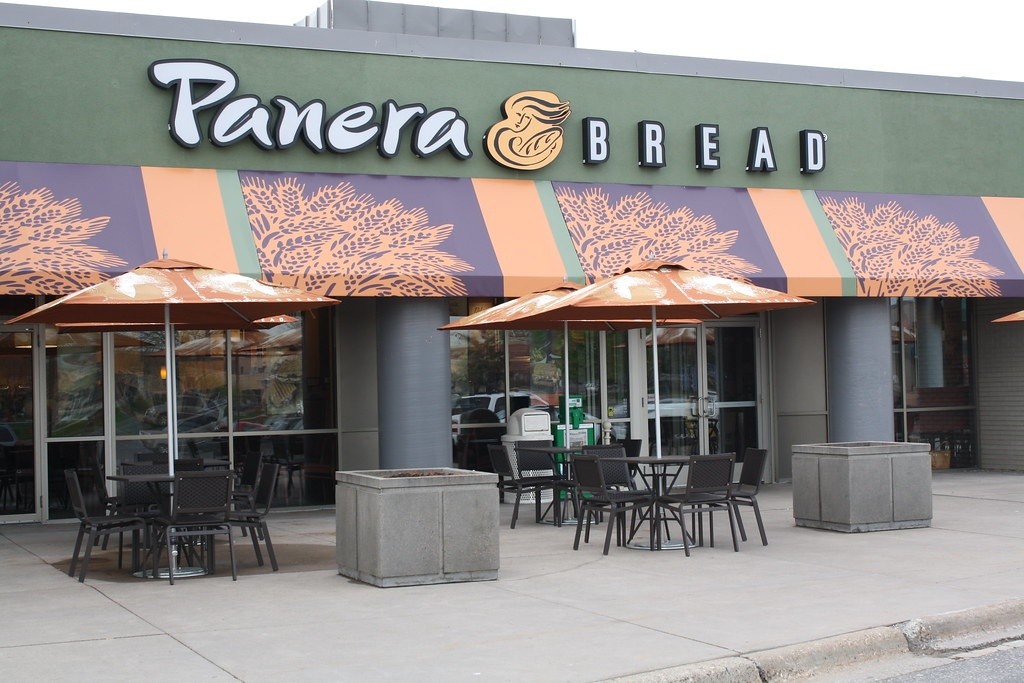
xmin=107 ymin=472 xmax=209 ymax=579
xmin=599 ymin=456 xmax=696 ymax=546
xmin=514 ymin=447 xmax=583 ymax=523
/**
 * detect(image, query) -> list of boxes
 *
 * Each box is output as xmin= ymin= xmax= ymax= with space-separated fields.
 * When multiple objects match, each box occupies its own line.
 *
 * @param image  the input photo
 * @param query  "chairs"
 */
xmin=581 ymin=444 xmax=653 ymax=547
xmin=572 ymin=454 xmax=656 ymax=556
xmin=173 ymin=458 xmax=203 ymax=474
xmin=140 ymin=469 xmax=237 ymax=584
xmin=616 ymin=438 xmax=642 ymax=478
xmin=710 ymin=447 xmax=768 ymax=547
xmin=63 ymin=468 xmax=160 ymax=584
xmin=121 ymin=462 xmax=171 ymax=511
xmin=513 ymin=439 xmax=556 ymax=523
xmin=487 ymin=443 xmax=558 ymax=529
xmin=206 ymin=463 xmax=281 ymax=575
xmin=656 ymin=452 xmax=740 ymax=557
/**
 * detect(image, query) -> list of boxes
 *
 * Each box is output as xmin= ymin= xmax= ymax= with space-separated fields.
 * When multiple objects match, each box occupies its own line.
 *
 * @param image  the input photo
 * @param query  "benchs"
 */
xmin=907 ymin=387 xmax=974 ymax=436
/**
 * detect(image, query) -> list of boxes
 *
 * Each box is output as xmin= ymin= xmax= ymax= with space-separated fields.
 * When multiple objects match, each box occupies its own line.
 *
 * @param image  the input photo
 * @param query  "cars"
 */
xmin=452 ymin=389 xmax=549 ymax=445
xmin=138 ymin=393 xmax=304 ymax=455
xmin=495 ymin=405 xmax=618 ymax=446
